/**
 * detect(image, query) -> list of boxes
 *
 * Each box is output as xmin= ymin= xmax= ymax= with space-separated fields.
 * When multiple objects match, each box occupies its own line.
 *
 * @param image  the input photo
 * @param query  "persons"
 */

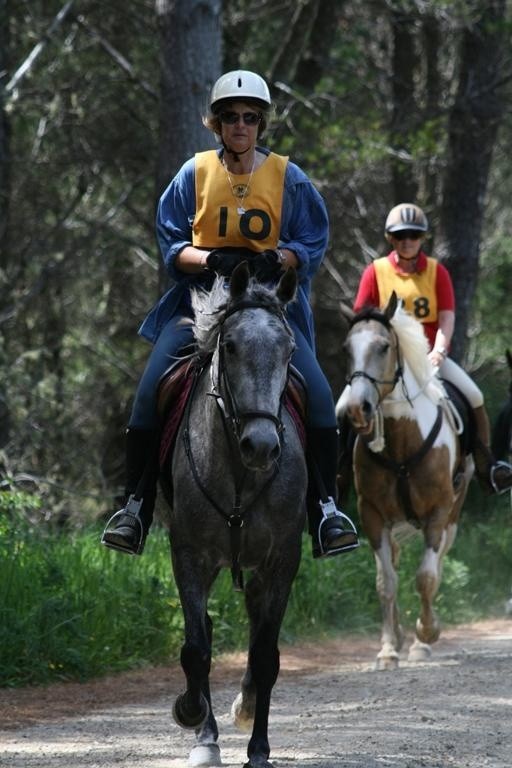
xmin=101 ymin=68 xmax=359 ymax=557
xmin=333 ymin=200 xmax=511 ymax=494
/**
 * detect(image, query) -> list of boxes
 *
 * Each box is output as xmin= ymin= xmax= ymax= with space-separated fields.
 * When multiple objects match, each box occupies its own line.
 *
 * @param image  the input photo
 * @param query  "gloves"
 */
xmin=204 ymin=250 xmax=240 ymax=277
xmin=244 ymin=249 xmax=284 ymax=285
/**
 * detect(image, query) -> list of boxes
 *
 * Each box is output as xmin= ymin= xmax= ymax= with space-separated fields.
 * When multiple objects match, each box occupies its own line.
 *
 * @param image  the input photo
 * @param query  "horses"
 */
xmin=149 ymin=259 xmax=309 ymax=768
xmin=346 ymin=289 xmax=477 ymax=672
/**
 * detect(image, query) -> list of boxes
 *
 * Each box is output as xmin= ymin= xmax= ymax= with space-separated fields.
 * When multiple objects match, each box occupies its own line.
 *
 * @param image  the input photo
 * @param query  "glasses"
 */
xmin=218 ymin=109 xmax=260 ymax=126
xmin=391 ymin=230 xmax=423 ymax=241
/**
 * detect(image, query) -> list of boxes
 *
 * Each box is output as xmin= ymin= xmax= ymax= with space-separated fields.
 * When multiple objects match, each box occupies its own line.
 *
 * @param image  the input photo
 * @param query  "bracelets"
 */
xmin=275 ymin=250 xmax=289 ymax=266
xmin=198 ymin=250 xmax=210 ymax=269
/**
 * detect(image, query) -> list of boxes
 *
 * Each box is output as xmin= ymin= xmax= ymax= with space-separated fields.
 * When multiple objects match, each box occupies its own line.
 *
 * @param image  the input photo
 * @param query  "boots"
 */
xmin=105 ymin=424 xmax=162 ymax=555
xmin=304 ymin=425 xmax=360 ymax=559
xmin=472 ymin=405 xmax=511 ymax=498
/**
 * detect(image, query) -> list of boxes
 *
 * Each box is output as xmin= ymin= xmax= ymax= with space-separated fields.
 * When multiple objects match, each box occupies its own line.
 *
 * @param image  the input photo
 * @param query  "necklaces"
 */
xmin=219 ymin=150 xmax=258 ymax=215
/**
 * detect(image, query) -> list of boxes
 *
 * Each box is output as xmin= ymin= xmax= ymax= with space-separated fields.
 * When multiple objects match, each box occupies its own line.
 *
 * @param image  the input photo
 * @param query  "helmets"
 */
xmin=207 ymin=69 xmax=273 ymax=111
xmin=383 ymin=202 xmax=429 ymax=234
